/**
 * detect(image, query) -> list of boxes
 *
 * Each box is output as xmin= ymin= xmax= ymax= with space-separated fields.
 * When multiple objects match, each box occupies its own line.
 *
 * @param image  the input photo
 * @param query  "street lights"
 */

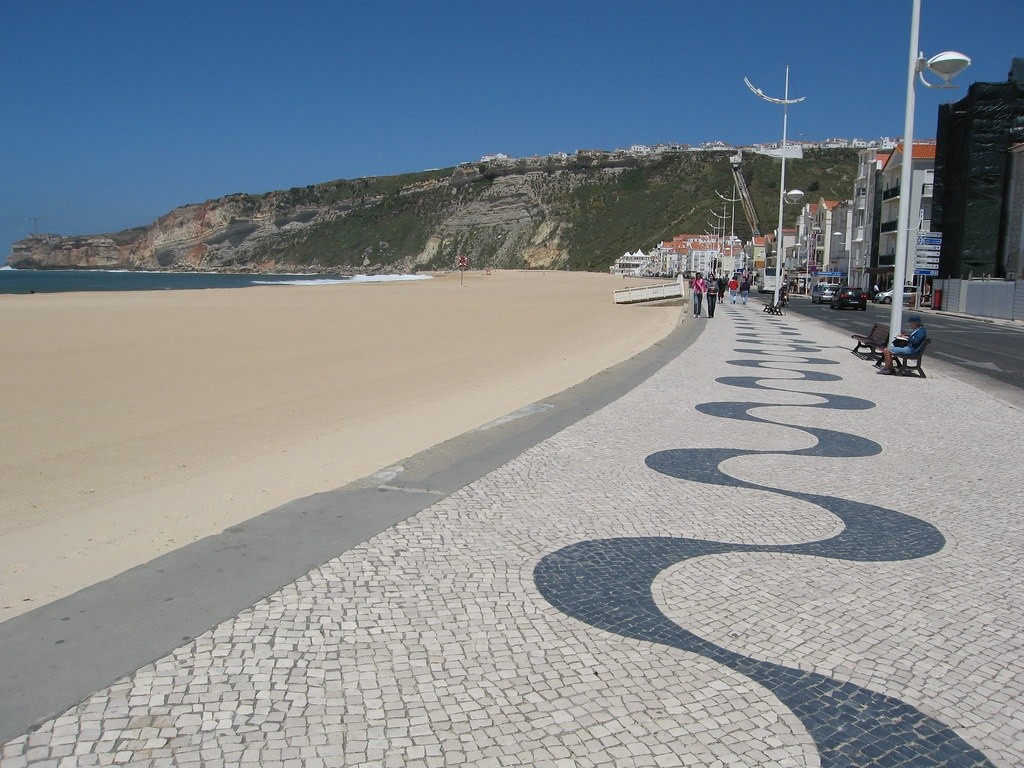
xmin=815 ymin=232 xmax=842 ymax=266
xmin=887 ymin=0 xmax=973 ymax=346
xmin=744 ymin=65 xmax=804 ymax=308
xmin=698 ymin=185 xmax=742 ymax=303
xmin=847 ymin=238 xmax=863 ymax=286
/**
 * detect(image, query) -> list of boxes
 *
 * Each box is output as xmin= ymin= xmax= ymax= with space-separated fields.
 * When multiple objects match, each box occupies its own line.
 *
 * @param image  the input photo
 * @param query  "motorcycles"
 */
xmin=781 ymin=289 xmax=790 ymax=307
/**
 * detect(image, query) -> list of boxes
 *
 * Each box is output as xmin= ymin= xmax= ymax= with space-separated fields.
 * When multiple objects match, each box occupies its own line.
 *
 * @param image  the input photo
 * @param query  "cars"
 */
xmin=830 ymin=287 xmax=867 ymax=311
xmin=874 ymin=285 xmax=923 ymax=303
xmin=812 ymin=284 xmax=842 ymax=304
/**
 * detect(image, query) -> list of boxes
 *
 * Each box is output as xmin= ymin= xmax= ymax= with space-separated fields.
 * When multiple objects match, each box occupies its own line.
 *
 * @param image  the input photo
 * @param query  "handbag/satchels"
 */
xmin=892 ymin=339 xmax=907 ymax=348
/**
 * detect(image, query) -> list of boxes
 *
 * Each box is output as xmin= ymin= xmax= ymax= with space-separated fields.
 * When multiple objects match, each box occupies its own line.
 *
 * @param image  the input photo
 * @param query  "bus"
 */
xmin=684 ymin=270 xmax=697 ymax=279
xmin=715 ymin=266 xmax=783 ymax=294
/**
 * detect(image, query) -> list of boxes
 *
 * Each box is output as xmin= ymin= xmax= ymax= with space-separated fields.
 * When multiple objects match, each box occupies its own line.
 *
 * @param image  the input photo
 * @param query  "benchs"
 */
xmin=852 ymin=323 xmax=931 ymax=379
xmin=762 ymin=299 xmax=784 ymax=315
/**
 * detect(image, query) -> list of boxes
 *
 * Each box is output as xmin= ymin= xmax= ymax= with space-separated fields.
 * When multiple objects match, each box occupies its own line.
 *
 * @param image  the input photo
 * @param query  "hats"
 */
xmin=905 ymin=315 xmax=920 ymax=323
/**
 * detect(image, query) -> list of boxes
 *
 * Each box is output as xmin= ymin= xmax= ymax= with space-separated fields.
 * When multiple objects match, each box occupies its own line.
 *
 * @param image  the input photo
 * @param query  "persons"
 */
xmin=740 ymin=278 xmax=750 ymax=305
xmin=717 ymin=278 xmax=725 ymax=304
xmin=706 ymin=274 xmax=718 ymax=318
xmin=872 ymin=283 xmax=880 ymax=303
xmin=727 ymin=276 xmax=739 ymax=304
xmin=876 ymin=313 xmax=926 ymax=375
xmin=692 ymin=272 xmax=706 ymax=318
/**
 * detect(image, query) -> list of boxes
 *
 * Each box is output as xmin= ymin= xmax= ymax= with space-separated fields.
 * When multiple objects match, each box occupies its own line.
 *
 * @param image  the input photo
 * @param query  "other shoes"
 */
xmin=890 ymin=369 xmax=895 ymax=375
xmin=694 ymin=314 xmax=700 ymax=318
xmin=877 ymin=369 xmax=890 ymax=374
